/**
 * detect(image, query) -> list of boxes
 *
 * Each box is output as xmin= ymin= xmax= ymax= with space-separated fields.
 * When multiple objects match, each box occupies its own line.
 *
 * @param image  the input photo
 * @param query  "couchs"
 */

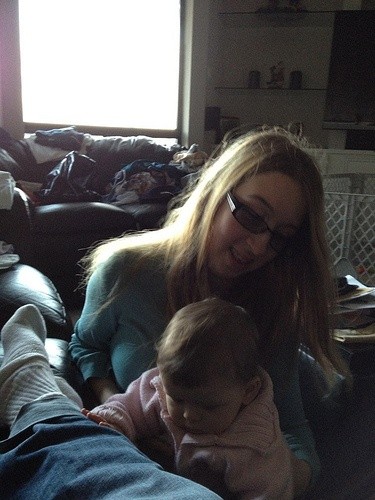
xmin=0 ymin=128 xmax=375 ymax=449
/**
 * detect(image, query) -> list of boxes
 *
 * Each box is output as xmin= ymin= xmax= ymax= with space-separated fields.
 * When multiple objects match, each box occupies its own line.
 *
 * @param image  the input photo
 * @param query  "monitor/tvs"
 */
xmin=322 ymin=10 xmax=375 ymax=131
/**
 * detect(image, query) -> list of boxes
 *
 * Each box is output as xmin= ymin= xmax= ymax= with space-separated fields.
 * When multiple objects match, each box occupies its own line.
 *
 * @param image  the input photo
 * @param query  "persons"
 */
xmin=80 ymin=296 xmax=295 ymax=499
xmin=67 ymin=121 xmax=337 ymax=500
xmin=0 ymin=303 xmax=224 ymax=500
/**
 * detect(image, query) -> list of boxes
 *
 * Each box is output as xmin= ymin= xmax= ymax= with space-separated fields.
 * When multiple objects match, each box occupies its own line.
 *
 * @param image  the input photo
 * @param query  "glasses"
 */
xmin=228 ymin=193 xmax=304 ymax=262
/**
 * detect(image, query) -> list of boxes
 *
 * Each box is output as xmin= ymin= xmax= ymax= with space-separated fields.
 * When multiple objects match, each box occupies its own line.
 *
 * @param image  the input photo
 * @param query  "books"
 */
xmin=328 ymin=324 xmax=375 ymax=345
